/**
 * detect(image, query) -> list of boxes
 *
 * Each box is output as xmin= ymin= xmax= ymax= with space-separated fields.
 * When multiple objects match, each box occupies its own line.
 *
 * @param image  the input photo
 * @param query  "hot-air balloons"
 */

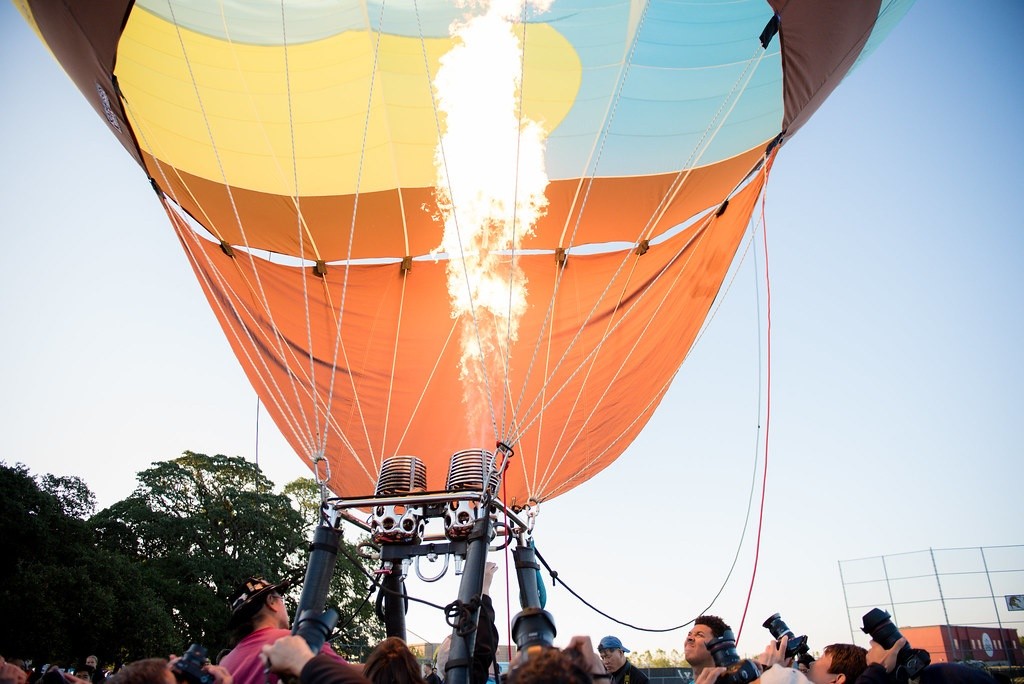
xmin=11 ymin=0 xmax=915 ymax=684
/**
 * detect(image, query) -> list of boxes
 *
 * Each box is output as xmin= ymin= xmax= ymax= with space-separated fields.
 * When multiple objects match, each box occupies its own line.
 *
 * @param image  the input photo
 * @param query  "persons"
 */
xmin=0 ymin=635 xmax=612 ymax=684
xmin=694 ymin=635 xmax=1012 ymax=684
xmin=684 ymin=615 xmax=732 ymax=684
xmin=597 ymin=635 xmax=651 ymax=684
xmin=214 ymin=576 xmax=346 ymax=684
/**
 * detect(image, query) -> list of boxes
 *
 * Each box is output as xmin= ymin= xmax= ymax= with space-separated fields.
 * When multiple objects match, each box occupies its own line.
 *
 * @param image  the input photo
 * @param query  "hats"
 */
xmin=225 ymin=576 xmax=290 ymax=621
xmin=597 ymin=636 xmax=630 ymax=654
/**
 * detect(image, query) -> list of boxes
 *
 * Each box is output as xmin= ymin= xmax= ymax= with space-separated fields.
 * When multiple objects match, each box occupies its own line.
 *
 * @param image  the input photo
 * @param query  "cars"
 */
xmin=486 ymin=662 xmax=510 ymax=684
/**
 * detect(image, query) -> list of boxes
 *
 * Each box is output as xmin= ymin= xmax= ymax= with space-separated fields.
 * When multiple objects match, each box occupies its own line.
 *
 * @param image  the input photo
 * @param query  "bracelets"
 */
xmin=591 ymin=672 xmax=612 ymax=680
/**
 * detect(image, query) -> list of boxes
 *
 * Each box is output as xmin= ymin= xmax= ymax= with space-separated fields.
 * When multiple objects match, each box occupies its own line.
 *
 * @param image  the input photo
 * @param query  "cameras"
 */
xmin=512 ymin=607 xmax=580 ymax=660
xmin=761 ymin=612 xmax=810 ymax=661
xmin=267 ymin=608 xmax=339 ymax=684
xmin=859 ymin=608 xmax=932 ymax=681
xmin=171 ymin=644 xmax=216 ymax=684
xmin=41 ymin=666 xmax=72 ymax=684
xmin=704 ymin=630 xmax=762 ymax=684
